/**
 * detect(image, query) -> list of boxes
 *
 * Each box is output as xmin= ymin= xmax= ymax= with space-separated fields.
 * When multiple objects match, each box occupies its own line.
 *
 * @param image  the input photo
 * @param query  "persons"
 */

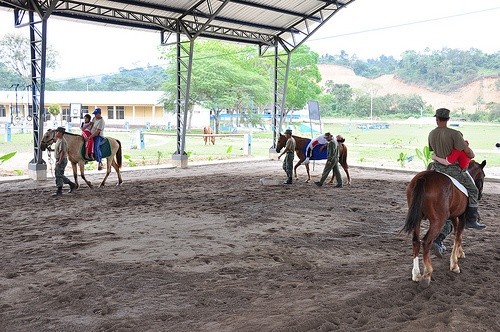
xmin=80 ymin=108 xmax=105 ymax=170
xmin=51 ymin=125 xmax=77 ymax=195
xmin=313 ymin=132 xmax=343 ymax=188
xmin=427 ymin=108 xmax=486 ymax=258
xmin=277 ymin=130 xmax=296 ymax=184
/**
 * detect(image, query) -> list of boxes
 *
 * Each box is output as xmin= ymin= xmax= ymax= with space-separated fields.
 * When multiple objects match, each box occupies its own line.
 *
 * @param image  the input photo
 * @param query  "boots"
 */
xmin=52 ymin=187 xmax=62 ymax=197
xmin=68 ymin=181 xmax=77 ymax=193
xmin=466 ymin=206 xmax=487 ymax=232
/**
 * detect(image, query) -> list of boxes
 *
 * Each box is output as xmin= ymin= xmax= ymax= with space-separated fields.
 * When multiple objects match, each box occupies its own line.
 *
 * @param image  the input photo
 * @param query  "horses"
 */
xmin=398 ymin=161 xmax=487 ymax=286
xmin=204 ymin=127 xmax=214 ymax=145
xmin=276 ymin=133 xmax=350 ymax=185
xmin=40 ymin=129 xmax=121 ymax=187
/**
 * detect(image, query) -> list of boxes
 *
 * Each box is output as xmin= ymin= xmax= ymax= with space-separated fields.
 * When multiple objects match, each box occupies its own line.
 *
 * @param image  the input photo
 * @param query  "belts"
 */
xmin=286 ymin=152 xmax=293 ymax=154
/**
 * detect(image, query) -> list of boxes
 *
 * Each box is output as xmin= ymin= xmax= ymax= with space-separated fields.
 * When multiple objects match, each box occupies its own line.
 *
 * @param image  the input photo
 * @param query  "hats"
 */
xmin=284 ymin=130 xmax=292 ymax=134
xmin=323 ymin=133 xmax=333 ymax=138
xmin=92 ymin=108 xmax=101 ymax=114
xmin=84 ymin=114 xmax=91 ymax=120
xmin=54 ymin=127 xmax=65 ymax=133
xmin=433 ymin=108 xmax=450 ymax=118
xmin=336 ymin=135 xmax=344 ymax=140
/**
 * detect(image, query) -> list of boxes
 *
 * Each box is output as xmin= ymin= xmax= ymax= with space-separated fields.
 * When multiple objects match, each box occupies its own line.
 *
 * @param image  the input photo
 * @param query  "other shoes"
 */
xmin=85 ymin=154 xmax=95 ymax=160
xmin=343 ymin=181 xmax=351 ymax=186
xmin=327 ymin=180 xmax=334 ymax=185
xmin=97 ymin=163 xmax=103 ymax=170
xmin=283 ymin=179 xmax=292 ymax=184
xmin=432 ymin=241 xmax=447 ymax=258
xmin=314 ymin=181 xmax=323 ymax=187
xmin=333 ymin=183 xmax=342 ymax=188
